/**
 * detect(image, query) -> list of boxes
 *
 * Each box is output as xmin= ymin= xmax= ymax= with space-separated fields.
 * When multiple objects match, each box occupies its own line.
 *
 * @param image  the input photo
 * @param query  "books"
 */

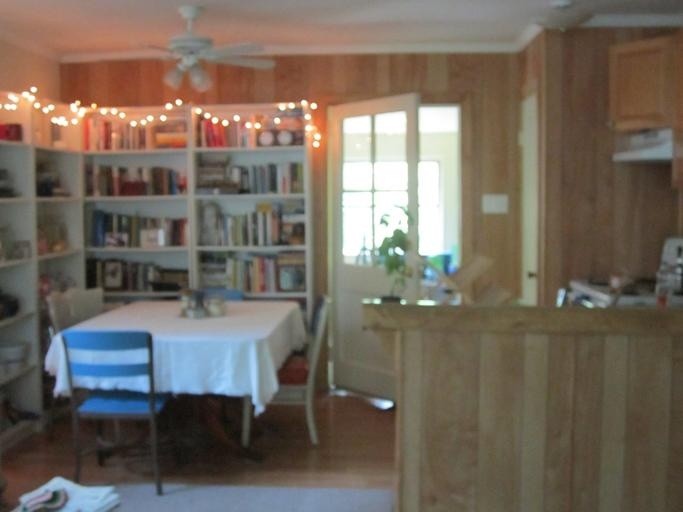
xmin=198 ymin=200 xmax=305 ymax=294
xmin=84 ymin=113 xmax=188 ymax=198
xmin=85 ymin=209 xmax=190 ymax=291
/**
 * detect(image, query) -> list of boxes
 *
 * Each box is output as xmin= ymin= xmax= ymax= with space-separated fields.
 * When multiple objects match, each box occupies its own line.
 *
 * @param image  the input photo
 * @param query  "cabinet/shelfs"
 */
xmin=190 ymin=101 xmax=315 ymax=300
xmin=607 ymin=34 xmax=682 ymax=135
xmin=81 ymin=106 xmax=190 ymax=302
xmin=33 ymin=97 xmax=83 ymax=307
xmin=0 ymin=89 xmax=41 ymax=454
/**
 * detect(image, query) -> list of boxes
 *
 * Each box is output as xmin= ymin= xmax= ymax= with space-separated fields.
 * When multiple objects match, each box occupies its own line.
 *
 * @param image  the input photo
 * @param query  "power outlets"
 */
xmin=162 ymin=55 xmax=212 ymax=94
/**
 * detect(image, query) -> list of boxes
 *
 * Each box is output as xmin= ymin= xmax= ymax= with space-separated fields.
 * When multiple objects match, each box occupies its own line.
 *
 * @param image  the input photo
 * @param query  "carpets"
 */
xmin=106 ymin=480 xmax=392 ymax=511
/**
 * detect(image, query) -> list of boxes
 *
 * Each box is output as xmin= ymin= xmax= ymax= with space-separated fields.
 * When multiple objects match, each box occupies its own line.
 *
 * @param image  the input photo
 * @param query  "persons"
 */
xmin=193 ymin=115 xmax=303 ymax=195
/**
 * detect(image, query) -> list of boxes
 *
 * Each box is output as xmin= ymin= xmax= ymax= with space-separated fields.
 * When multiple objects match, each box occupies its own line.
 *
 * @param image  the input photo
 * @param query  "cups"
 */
xmin=656 ymin=271 xmax=675 ymax=307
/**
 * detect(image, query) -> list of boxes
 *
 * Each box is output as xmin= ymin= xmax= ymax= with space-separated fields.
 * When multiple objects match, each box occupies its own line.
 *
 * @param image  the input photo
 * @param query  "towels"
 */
xmin=19 ymin=474 xmax=119 ymax=511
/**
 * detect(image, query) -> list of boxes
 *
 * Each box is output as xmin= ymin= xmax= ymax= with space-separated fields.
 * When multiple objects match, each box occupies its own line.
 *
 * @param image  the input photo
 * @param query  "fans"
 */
xmin=147 ymin=4 xmax=275 ymax=74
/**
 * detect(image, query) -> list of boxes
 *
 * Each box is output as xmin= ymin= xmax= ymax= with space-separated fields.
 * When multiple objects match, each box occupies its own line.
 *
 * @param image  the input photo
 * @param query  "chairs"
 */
xmin=242 ymin=295 xmax=331 ymax=451
xmin=46 ymin=286 xmax=102 ymax=340
xmin=60 ymin=329 xmax=170 ymax=493
